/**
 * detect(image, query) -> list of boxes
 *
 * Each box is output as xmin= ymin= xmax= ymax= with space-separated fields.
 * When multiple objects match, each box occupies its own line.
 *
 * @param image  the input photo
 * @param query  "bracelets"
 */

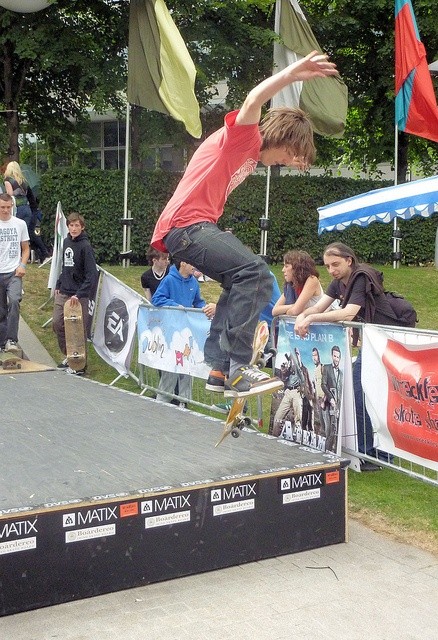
xmin=19 ymin=263 xmax=28 ymax=270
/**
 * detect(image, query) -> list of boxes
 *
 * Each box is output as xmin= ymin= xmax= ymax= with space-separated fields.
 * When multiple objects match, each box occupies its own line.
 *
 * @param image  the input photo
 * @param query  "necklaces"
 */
xmin=150 ymin=265 xmax=168 ymax=281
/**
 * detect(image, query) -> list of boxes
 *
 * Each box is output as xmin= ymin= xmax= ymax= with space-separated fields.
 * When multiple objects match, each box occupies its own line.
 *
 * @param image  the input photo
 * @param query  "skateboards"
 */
xmin=63 ymin=299 xmax=87 ymax=371
xmin=214 ymin=320 xmax=276 ymax=450
xmin=0 ymin=349 xmax=22 ymax=369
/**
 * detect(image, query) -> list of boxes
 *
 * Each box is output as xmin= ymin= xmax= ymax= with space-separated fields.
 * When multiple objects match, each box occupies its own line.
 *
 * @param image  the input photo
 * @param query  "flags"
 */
xmin=127 ymin=0 xmax=203 ymax=142
xmin=270 ymin=0 xmax=351 ymax=142
xmin=392 ymin=0 xmax=438 ymax=143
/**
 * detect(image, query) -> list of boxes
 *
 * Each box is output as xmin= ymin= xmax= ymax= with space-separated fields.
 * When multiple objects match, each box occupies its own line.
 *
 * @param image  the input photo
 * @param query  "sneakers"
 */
xmin=65 ymin=368 xmax=86 ymax=375
xmin=205 ymin=370 xmax=230 ymax=392
xmin=56 ymin=356 xmax=70 ymax=370
xmin=5 ymin=339 xmax=18 ymax=352
xmin=223 ymin=366 xmax=283 ymax=398
xmin=38 ymin=256 xmax=52 ymax=268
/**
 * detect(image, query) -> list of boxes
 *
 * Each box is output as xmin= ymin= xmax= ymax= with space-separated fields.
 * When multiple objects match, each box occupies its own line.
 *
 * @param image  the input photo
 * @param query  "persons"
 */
xmin=3 ymin=161 xmax=34 ymax=265
xmin=266 ymin=248 xmax=334 ymax=319
xmin=271 ymin=344 xmax=345 ymax=455
xmin=22 ymin=180 xmax=54 ymax=268
xmin=0 ymin=192 xmax=32 ymax=356
xmin=50 ymin=211 xmax=100 ymax=377
xmin=200 ymin=251 xmax=283 ymax=419
xmin=147 ymin=254 xmax=209 ymax=409
xmin=146 ymin=49 xmax=342 ymax=398
xmin=289 ymin=240 xmax=398 ymax=475
xmin=141 ymin=241 xmax=186 ymax=408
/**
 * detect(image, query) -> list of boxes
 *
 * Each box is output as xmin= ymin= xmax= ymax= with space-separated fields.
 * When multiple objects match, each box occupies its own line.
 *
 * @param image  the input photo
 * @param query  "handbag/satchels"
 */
xmin=386 ymin=290 xmax=417 ymax=327
